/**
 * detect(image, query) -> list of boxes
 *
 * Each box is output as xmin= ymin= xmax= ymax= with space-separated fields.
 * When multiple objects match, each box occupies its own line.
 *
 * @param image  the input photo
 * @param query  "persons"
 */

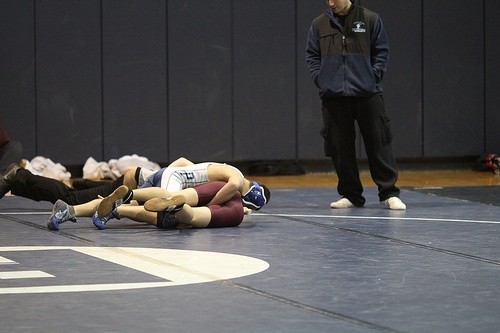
xmin=304 ymin=0 xmax=407 ymax=211
xmin=0 ymin=153 xmax=272 ymax=230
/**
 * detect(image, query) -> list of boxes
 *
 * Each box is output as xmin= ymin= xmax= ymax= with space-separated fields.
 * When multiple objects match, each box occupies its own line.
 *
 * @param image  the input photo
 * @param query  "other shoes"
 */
xmin=91 ymin=207 xmax=121 ymax=230
xmin=330 ymin=198 xmax=354 ymax=208
xmin=144 ymin=194 xmax=186 ymax=212
xmin=47 ymin=199 xmax=77 ymax=231
xmin=0 ymin=163 xmax=21 ymax=200
xmin=71 ymin=177 xmax=90 ymax=189
xmin=380 ymin=196 xmax=407 ymax=209
xmin=97 ymin=184 xmax=129 ymax=218
